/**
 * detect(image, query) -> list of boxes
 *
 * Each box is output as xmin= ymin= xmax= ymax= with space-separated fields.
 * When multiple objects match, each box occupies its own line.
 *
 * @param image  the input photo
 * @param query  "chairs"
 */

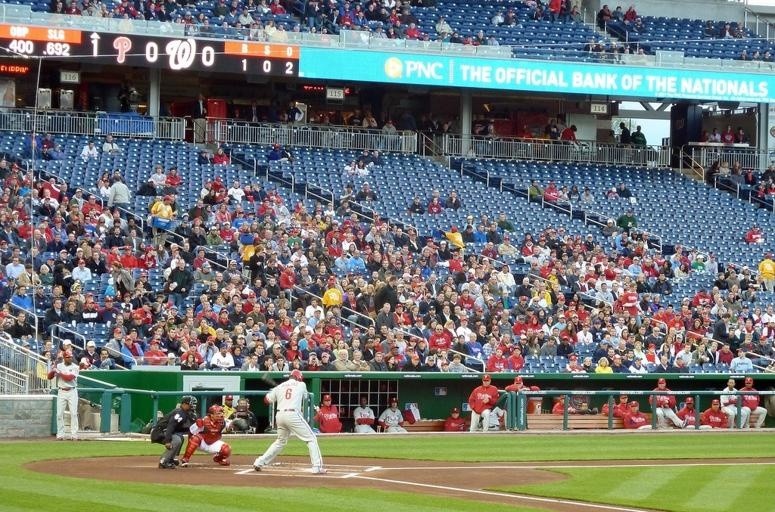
xmin=1 ymin=0 xmax=775 ymax=51
xmin=0 ymin=107 xmax=774 ymax=377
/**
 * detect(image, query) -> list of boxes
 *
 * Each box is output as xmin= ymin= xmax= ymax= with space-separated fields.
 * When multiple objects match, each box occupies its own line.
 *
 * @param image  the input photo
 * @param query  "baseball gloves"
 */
xmin=228 ymin=417 xmax=249 ymax=433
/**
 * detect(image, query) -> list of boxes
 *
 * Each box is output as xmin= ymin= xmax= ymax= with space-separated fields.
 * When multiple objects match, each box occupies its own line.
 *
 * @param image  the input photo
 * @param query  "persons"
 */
xmin=582 ymin=38 xmax=645 ymax=64
xmin=737 ymin=48 xmax=774 ymax=62
xmin=597 ymin=4 xmax=647 ymax=42
xmin=702 ymin=19 xmax=748 ymax=40
xmin=251 ymin=369 xmax=328 ymax=475
xmin=51 ymin=0 xmax=499 ymax=47
xmin=1 ymin=90 xmax=775 ymax=434
xmin=180 ymin=404 xmax=232 ymax=468
xmin=150 ymin=395 xmax=198 ymax=470
xmin=492 ymin=0 xmax=584 ymax=26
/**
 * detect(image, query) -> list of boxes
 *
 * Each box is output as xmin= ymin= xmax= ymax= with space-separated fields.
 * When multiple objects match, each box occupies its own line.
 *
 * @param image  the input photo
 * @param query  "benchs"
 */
xmin=386 ymin=420 xmax=447 ymax=431
xmin=523 ymin=413 xmax=768 ymax=429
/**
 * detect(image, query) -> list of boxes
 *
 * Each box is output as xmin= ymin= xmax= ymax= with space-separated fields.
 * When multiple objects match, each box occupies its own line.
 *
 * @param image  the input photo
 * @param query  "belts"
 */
xmin=59 ymin=387 xmax=75 ymax=390
xmin=276 ymin=409 xmax=295 ymax=411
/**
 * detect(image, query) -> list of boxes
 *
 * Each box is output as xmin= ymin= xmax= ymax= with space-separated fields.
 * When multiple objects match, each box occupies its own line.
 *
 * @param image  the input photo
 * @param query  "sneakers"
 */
xmin=312 ymin=469 xmax=326 ymax=474
xmin=159 ymin=456 xmax=230 ymax=468
xmin=252 ymin=464 xmax=260 ymax=471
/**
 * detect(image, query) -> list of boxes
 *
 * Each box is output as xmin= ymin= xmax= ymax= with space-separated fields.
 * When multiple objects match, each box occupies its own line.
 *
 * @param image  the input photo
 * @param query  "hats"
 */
xmin=323 ymin=395 xmax=331 ymax=400
xmin=389 ymin=396 xmax=397 ymax=402
xmin=63 ymin=340 xmax=96 ymax=357
xmin=483 ymin=374 xmax=490 ymax=381
xmin=515 ymin=377 xmax=521 ymax=382
xmin=225 ymin=396 xmax=232 ymax=400
xmin=451 ymin=407 xmax=459 ymax=413
xmin=608 ymin=377 xmax=753 ymax=407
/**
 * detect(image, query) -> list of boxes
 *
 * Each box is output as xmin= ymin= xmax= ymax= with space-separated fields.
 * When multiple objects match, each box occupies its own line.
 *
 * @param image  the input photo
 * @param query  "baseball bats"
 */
xmin=51 ymin=354 xmax=58 ymax=376
xmin=261 ymin=374 xmax=280 ymax=386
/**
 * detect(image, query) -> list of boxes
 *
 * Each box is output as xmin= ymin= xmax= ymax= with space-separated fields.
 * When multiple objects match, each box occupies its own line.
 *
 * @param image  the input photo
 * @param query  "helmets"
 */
xmin=291 ymin=369 xmax=303 ymax=381
xmin=182 ymin=396 xmax=197 ymax=416
xmin=209 ymin=405 xmax=224 ymax=424
